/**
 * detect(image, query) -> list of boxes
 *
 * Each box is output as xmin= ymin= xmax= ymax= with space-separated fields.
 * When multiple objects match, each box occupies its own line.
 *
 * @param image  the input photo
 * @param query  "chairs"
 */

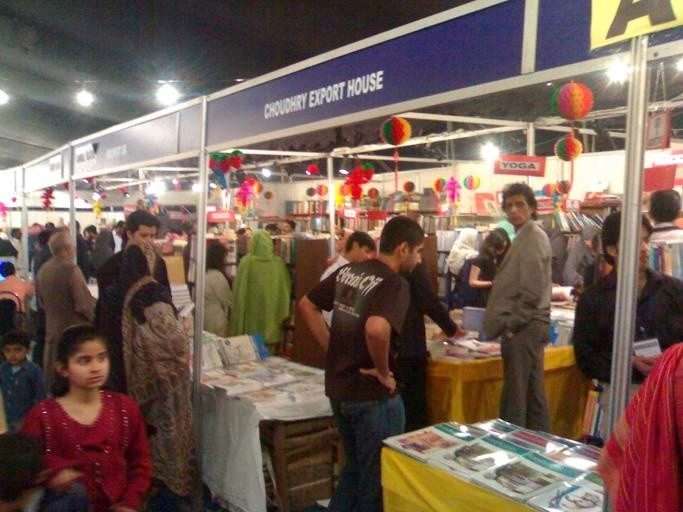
xmin=0 ymin=291 xmax=30 ymax=336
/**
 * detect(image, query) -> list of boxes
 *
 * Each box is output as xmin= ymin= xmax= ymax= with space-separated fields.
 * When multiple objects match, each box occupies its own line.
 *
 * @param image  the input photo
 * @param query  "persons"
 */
xmin=1 ymin=183 xmax=682 ymax=512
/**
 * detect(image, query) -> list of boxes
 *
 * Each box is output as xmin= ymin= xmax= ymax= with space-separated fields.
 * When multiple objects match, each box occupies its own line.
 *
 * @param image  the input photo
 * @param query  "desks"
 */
xmin=380 ymin=420 xmax=606 ymax=512
xmin=423 ymin=321 xmax=599 ymax=441
xmin=193 ymin=354 xmax=348 ymax=512
